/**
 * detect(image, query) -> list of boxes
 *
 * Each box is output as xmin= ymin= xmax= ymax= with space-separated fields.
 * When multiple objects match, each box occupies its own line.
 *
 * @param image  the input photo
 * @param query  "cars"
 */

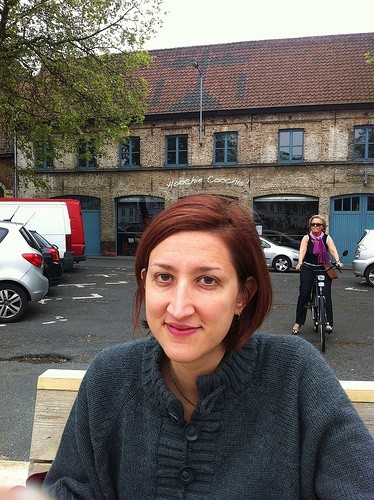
xmin=261 ymin=230 xmax=305 ymax=249
xmin=259 ymin=237 xmax=300 ymax=272
xmin=27 ymin=230 xmax=63 ymax=287
xmin=352 ymin=229 xmax=374 ymax=285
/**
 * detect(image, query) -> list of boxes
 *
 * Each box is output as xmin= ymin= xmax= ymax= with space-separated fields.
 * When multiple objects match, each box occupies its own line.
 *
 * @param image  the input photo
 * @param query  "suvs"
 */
xmin=0 ymin=222 xmax=50 ymax=322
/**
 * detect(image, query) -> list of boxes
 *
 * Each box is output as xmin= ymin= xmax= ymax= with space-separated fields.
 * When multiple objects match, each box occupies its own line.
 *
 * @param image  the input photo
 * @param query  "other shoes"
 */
xmin=325 ymin=321 xmax=333 ymax=332
xmin=292 ymin=322 xmax=302 ymax=333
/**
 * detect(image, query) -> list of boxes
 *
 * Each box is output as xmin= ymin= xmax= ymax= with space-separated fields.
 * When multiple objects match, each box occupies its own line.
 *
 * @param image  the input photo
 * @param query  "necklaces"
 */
xmin=168 ymin=361 xmax=195 ymax=407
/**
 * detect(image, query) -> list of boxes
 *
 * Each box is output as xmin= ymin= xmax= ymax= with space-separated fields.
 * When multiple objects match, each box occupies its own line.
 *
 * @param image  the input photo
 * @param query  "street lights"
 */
xmin=191 ymin=60 xmax=203 ymax=145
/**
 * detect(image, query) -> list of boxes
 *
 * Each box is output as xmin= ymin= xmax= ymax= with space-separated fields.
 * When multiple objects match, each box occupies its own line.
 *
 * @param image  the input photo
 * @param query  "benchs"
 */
xmin=29 ymin=369 xmax=374 ymax=479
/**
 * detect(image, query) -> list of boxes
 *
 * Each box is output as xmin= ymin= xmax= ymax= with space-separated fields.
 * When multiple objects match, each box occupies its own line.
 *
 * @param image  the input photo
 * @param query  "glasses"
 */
xmin=310 ymin=223 xmax=324 ymax=227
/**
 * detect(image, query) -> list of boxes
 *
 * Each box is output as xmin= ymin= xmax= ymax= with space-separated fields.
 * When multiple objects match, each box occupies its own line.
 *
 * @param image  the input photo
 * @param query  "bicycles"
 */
xmin=304 ymin=249 xmax=349 ymax=352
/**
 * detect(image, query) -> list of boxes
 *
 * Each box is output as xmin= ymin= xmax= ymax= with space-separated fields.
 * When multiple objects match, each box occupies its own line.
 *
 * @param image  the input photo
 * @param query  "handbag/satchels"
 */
xmin=323 ymin=263 xmax=339 ymax=279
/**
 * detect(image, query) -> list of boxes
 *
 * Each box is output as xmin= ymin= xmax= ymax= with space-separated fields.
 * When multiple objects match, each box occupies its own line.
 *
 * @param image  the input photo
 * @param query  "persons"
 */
xmin=40 ymin=195 xmax=374 ymax=500
xmin=292 ymin=215 xmax=343 ymax=335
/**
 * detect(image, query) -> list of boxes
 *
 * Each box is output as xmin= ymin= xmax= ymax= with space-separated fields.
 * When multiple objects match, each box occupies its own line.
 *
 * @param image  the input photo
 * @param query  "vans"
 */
xmin=0 ymin=198 xmax=90 ymax=260
xmin=0 ymin=201 xmax=73 ymax=272
